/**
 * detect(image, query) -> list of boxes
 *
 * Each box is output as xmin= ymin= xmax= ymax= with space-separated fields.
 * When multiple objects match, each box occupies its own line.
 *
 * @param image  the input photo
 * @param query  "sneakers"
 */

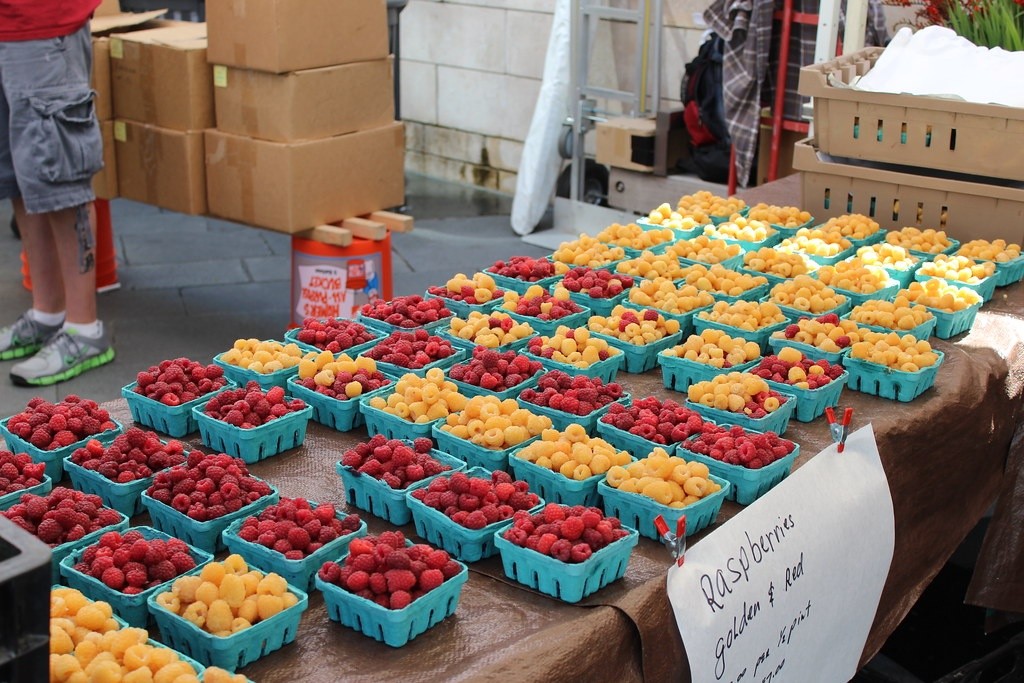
xmin=0 ymin=311 xmax=115 ymax=386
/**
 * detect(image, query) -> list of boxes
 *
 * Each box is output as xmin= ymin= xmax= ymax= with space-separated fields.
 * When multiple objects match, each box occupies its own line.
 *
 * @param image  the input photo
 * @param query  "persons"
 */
xmin=0 ymin=0 xmax=117 ymax=386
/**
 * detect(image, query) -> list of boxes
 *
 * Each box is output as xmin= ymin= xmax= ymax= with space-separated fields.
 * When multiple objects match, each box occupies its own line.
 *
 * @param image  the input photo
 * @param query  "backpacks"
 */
xmin=680 ymin=32 xmax=755 ymax=187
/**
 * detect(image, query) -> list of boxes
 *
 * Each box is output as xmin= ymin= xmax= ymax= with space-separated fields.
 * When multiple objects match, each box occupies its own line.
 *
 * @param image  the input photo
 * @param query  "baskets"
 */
xmin=0 ymin=203 xmax=1024 ymax=683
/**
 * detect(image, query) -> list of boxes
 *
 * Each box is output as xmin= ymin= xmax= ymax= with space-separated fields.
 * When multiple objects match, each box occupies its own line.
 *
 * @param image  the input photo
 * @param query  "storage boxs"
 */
xmin=741 ymin=363 xmax=850 ymax=423
xmin=594 ymin=115 xmax=657 ymax=171
xmin=545 ymin=250 xmax=633 ymax=276
xmin=596 ymin=194 xmax=782 ymax=258
xmin=792 ymin=45 xmax=1024 ymax=402
xmin=769 ymin=216 xmax=814 ymax=242
xmin=737 ymin=261 xmax=821 ymax=290
xmin=88 ymin=0 xmax=407 ymax=233
xmin=758 ymin=285 xmax=851 ymax=326
xmin=766 ymin=327 xmax=851 ymax=369
xmin=0 ymin=261 xmax=768 ymax=683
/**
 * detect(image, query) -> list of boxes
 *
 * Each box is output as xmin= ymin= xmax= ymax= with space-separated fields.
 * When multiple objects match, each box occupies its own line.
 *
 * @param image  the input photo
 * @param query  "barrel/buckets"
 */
xmin=20 ymin=196 xmax=121 ymax=293
xmin=286 ymin=230 xmax=392 ymax=337
